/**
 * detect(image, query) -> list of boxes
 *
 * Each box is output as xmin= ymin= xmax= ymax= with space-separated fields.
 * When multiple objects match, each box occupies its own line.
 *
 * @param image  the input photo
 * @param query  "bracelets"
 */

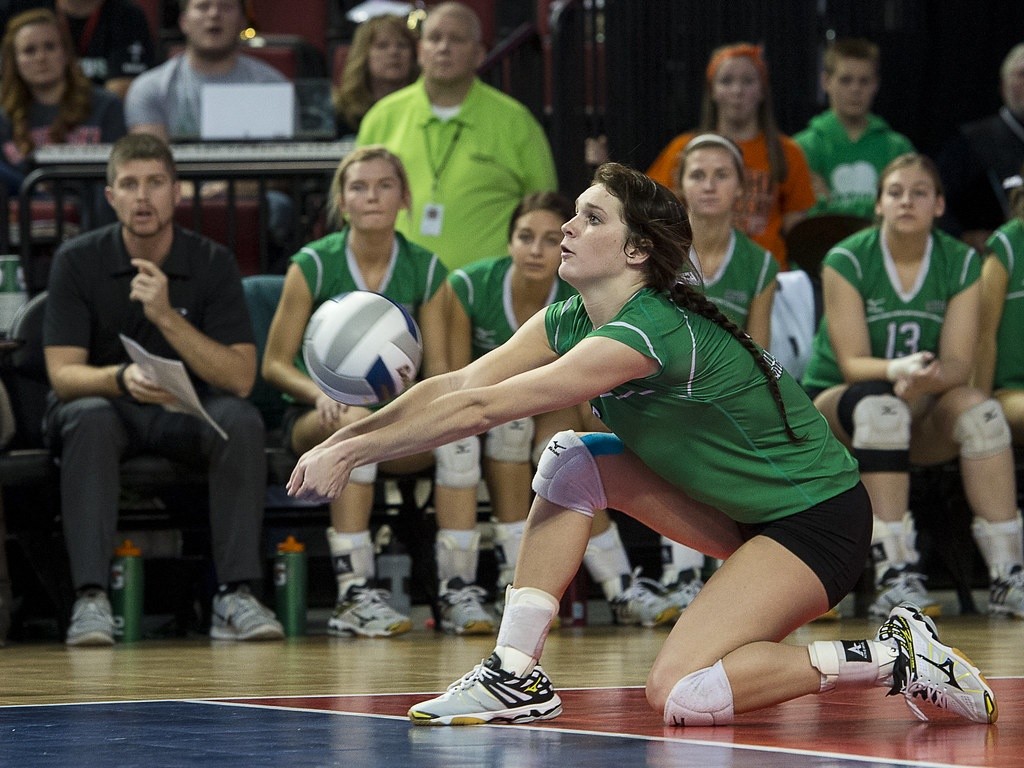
xmin=115 ymin=364 xmax=132 ymax=397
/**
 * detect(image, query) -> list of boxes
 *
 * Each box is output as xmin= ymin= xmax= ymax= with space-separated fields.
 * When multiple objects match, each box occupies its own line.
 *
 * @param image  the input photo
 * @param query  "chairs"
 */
xmin=0 ymin=274 xmax=528 ymax=646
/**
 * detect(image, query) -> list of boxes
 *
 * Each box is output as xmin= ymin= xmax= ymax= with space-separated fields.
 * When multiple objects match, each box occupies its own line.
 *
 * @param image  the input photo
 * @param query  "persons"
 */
xmin=286 ymin=162 xmax=999 ymax=731
xmin=0 ymin=0 xmax=1024 ymax=643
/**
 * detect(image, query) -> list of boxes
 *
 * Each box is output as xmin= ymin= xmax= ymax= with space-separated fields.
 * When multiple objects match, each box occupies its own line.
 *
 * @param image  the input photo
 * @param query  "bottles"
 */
xmin=273 ymin=535 xmax=304 ymax=637
xmin=109 ymin=539 xmax=144 ymax=643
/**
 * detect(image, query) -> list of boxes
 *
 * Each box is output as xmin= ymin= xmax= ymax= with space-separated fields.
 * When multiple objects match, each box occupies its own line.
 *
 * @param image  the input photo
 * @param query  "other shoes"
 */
xmin=662 ymin=569 xmax=705 ymax=612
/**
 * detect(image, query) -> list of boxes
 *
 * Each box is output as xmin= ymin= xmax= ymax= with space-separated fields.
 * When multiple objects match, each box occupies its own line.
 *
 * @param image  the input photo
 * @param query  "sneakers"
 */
xmin=209 ymin=587 xmax=284 ymax=641
xmin=65 ymin=587 xmax=125 ymax=647
xmin=598 ymin=566 xmax=681 ymax=628
xmin=868 ymin=563 xmax=942 ymax=618
xmin=894 ymin=720 xmax=998 ymax=765
xmin=873 ymin=601 xmax=998 ymax=725
xmin=438 ymin=575 xmax=493 ymax=635
xmin=408 ymin=725 xmax=563 ymax=768
xmin=327 ymin=577 xmax=414 ymax=638
xmin=494 ymin=567 xmax=563 ymax=629
xmin=985 ymin=563 xmax=1024 ymax=619
xmin=406 ymin=652 xmax=564 ymax=725
